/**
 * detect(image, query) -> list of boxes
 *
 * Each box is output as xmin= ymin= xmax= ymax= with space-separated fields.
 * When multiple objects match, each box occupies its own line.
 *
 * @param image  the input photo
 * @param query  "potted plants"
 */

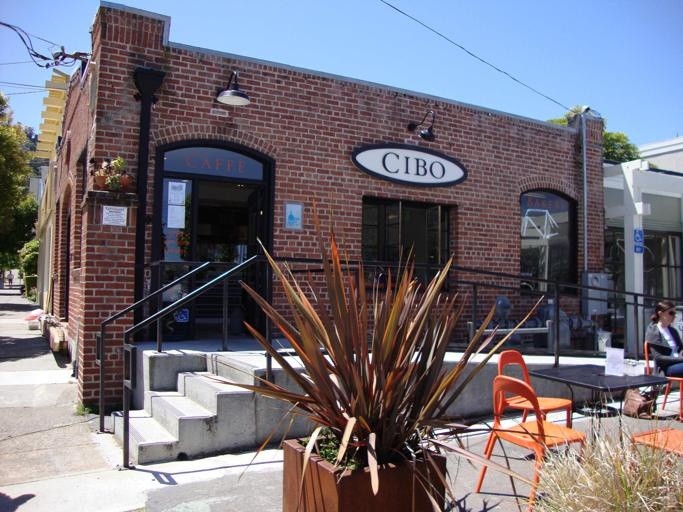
xmin=200 ymin=194 xmax=545 ymax=511
xmin=93 ymin=155 xmax=133 ymax=193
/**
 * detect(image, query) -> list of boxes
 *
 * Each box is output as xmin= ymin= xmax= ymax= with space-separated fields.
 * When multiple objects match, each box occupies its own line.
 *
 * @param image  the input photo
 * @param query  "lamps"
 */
xmin=216 ymin=69 xmax=251 ymax=106
xmin=407 ymin=111 xmax=436 ymax=141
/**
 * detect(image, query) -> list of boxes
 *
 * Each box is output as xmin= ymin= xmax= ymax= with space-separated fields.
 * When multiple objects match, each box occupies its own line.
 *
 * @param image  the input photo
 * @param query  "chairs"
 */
xmin=475 ymin=349 xmax=587 ymax=512
xmin=644 ymin=340 xmax=683 ymax=423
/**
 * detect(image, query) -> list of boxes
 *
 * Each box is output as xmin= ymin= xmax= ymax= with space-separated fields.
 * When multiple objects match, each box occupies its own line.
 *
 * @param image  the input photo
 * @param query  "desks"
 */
xmin=527 ymin=364 xmax=670 ymax=459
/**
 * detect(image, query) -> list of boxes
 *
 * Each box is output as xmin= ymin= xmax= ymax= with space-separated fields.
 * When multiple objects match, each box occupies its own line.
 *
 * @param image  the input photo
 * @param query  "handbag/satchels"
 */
xmin=623 ymin=385 xmax=659 ymax=419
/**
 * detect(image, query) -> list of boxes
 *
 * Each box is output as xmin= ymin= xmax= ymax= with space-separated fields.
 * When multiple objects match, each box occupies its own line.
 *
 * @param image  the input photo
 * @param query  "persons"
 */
xmin=645 ymin=301 xmax=683 ymax=378
xmin=7 ymin=271 xmax=14 ymax=288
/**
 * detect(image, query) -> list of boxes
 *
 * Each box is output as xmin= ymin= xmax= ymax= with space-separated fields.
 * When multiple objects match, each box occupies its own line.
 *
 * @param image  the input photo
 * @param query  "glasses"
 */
xmin=668 ymin=311 xmax=676 ymax=316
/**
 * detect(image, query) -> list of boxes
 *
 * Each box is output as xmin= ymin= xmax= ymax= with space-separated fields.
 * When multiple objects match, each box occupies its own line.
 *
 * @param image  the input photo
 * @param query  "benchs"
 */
xmin=467 ymin=321 xmax=554 ymax=353
